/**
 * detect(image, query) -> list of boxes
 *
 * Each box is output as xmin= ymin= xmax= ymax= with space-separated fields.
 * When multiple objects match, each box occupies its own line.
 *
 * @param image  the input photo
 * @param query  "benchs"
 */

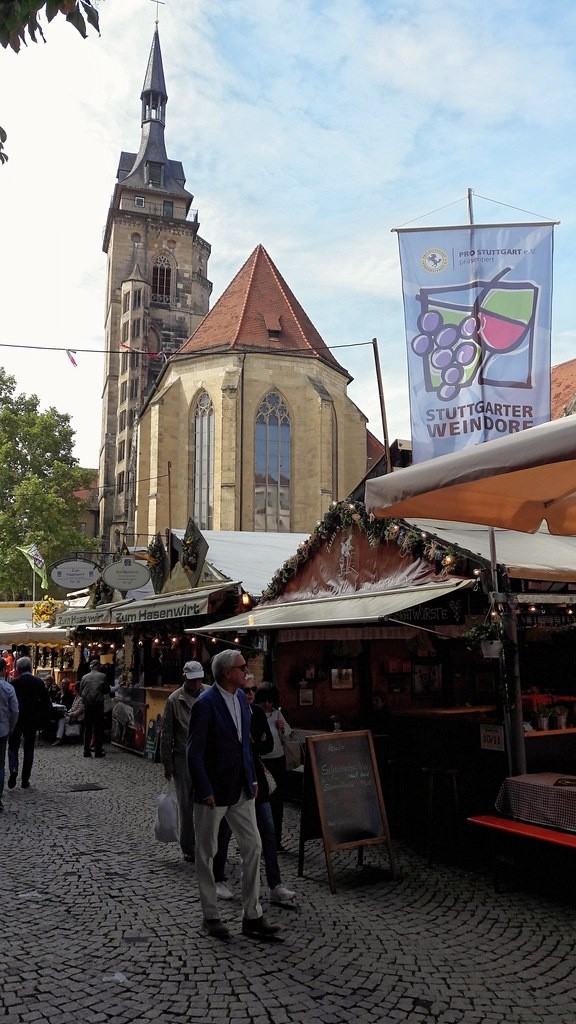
xmin=467 ymin=815 xmax=576 ymax=850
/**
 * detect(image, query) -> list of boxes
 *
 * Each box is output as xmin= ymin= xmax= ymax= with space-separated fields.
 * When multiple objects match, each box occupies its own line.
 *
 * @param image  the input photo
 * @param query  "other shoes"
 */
xmin=275 ymin=844 xmax=285 ymax=852
xmin=83 ymin=753 xmax=92 ymax=758
xmin=52 ymin=739 xmax=61 ymax=746
xmin=183 ymin=849 xmax=195 ymax=863
xmin=94 ymin=752 xmax=106 ymax=758
xmin=7 ymin=768 xmax=18 ymax=789
xmin=201 ymin=919 xmax=229 ymax=939
xmin=21 ymin=782 xmax=30 ymax=789
xmin=241 ymin=915 xmax=281 ymax=936
xmin=0 ymin=801 xmax=4 ymax=809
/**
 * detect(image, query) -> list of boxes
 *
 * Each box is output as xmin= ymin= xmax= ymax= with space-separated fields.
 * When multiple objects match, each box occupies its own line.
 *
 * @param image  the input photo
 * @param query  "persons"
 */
xmin=42 ymin=676 xmax=85 ymax=748
xmin=0 ymin=650 xmax=21 ymax=678
xmin=211 ymin=669 xmax=300 ymax=900
xmin=8 ymin=656 xmax=50 ymax=789
xmin=158 ymin=659 xmax=217 ymax=860
xmin=0 ymin=657 xmax=20 ymax=811
xmin=80 ymin=661 xmax=110 ymax=757
xmin=186 ymin=649 xmax=283 ymax=943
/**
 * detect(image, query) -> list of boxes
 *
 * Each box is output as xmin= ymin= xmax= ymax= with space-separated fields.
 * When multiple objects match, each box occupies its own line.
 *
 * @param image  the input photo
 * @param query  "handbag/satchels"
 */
xmin=65 ymin=717 xmax=80 ymax=736
xmin=283 ymin=740 xmax=301 ymax=769
xmin=155 ymin=778 xmax=179 ymax=843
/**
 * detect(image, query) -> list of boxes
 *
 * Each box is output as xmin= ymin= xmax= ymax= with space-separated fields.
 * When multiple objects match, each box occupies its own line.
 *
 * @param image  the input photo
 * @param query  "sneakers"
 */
xmin=215 ymin=883 xmax=233 ymax=900
xmin=269 ymin=883 xmax=298 ymax=899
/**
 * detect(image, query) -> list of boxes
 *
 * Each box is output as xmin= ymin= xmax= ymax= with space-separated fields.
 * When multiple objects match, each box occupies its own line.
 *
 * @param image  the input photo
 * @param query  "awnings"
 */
xmin=56 ymin=600 xmax=129 ymax=626
xmin=110 ymin=586 xmax=228 ymax=625
xmin=182 ymin=584 xmax=463 ymax=650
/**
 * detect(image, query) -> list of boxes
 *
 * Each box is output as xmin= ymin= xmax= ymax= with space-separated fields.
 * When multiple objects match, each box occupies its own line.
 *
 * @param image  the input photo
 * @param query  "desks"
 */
xmin=494 ymin=772 xmax=576 ymax=892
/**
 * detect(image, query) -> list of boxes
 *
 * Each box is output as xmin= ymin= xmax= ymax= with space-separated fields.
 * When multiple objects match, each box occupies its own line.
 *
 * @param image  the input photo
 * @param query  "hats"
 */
xmin=43 ymin=674 xmax=55 ymax=680
xmin=183 ymin=661 xmax=204 ymax=679
xmin=90 ymin=660 xmax=102 ymax=668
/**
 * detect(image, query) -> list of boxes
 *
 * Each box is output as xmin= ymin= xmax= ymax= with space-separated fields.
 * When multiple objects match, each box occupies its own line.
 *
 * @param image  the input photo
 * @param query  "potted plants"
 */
xmin=553 ymin=705 xmax=568 ymax=729
xmin=534 ymin=705 xmax=551 ymax=730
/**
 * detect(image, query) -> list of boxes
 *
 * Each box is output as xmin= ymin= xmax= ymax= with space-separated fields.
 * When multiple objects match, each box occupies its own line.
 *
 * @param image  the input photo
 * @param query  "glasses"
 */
xmin=231 ymin=663 xmax=248 ymax=671
xmin=241 ymin=686 xmax=258 ymax=694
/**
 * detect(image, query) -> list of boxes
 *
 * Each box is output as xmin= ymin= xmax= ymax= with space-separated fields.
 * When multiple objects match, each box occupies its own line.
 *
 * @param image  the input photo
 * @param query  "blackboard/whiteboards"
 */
xmin=299 ymin=729 xmax=392 ymax=851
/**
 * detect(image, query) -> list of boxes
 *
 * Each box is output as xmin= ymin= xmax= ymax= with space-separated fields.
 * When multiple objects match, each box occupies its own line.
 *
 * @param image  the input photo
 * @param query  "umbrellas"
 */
xmin=365 ymin=411 xmax=576 ymax=537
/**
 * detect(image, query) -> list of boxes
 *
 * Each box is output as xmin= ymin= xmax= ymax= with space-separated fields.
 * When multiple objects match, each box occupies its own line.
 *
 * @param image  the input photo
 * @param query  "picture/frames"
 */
xmin=411 ymin=656 xmax=441 ymax=698
xmin=298 ymin=689 xmax=314 ymax=705
xmin=330 ymin=667 xmax=354 ymax=691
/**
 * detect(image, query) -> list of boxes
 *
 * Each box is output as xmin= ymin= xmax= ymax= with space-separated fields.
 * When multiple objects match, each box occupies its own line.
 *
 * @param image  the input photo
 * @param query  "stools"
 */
xmin=421 ymin=766 xmax=464 ymax=867
